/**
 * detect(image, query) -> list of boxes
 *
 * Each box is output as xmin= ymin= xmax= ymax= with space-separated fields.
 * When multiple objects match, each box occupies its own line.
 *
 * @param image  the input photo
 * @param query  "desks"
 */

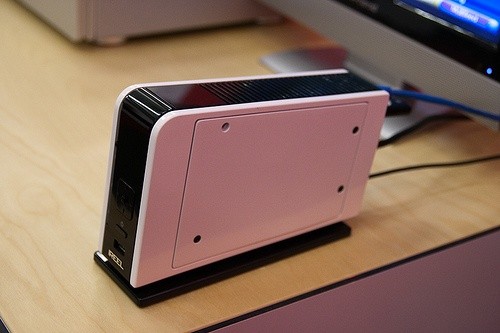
xmin=0 ymin=2 xmax=500 ymax=332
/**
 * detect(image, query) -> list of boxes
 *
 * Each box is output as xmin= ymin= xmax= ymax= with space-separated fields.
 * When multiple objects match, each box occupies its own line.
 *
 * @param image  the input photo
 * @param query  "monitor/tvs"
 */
xmin=340 ymin=0 xmax=500 ymax=80
